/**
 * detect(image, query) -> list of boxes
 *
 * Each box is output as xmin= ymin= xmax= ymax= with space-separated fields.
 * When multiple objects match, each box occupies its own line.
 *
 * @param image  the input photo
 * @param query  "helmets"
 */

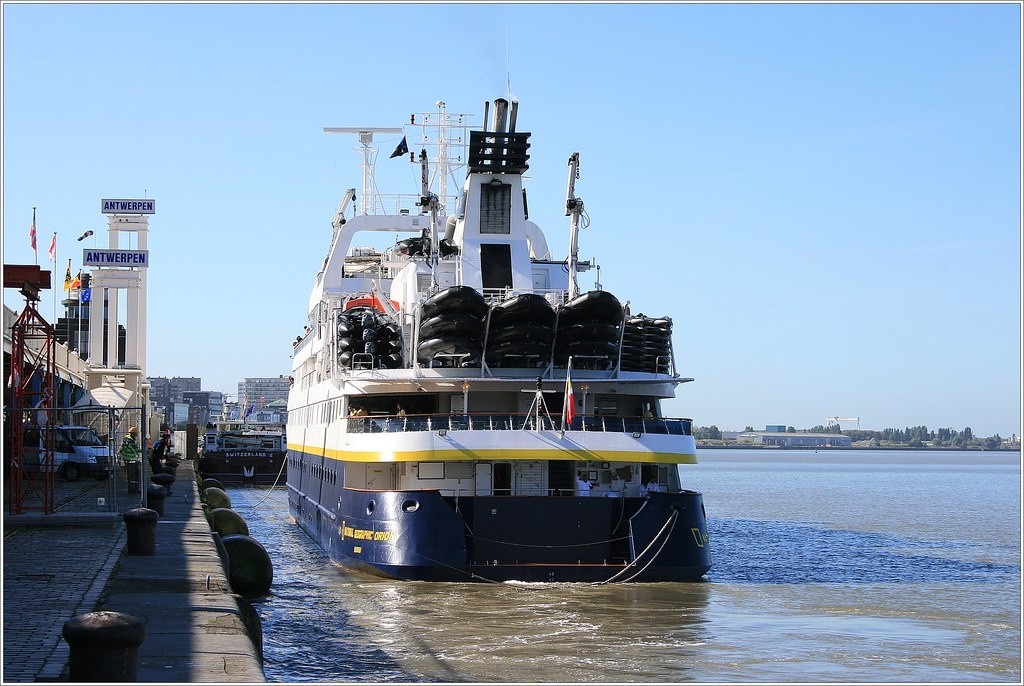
xmin=128 ymin=427 xmax=141 ymax=433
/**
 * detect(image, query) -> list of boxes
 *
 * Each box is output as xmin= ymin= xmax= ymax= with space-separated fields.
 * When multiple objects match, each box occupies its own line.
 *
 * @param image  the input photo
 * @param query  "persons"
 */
xmin=288 ymin=376 xmax=295 ymax=384
xmin=644 ymin=407 xmax=656 ymax=420
xmin=296 ymin=335 xmax=303 ymax=343
xmin=143 ymin=434 xmax=171 ymax=475
xmin=304 ymin=325 xmax=310 ymax=335
xmin=646 ymin=477 xmax=660 ymax=492
xmin=396 ymin=403 xmax=406 ymax=419
xmin=576 ymin=473 xmax=593 ymax=496
xmin=348 ymin=404 xmax=368 ymax=416
xmin=119 ymin=427 xmax=142 ymax=493
xmin=293 ymin=342 xmax=297 ymax=346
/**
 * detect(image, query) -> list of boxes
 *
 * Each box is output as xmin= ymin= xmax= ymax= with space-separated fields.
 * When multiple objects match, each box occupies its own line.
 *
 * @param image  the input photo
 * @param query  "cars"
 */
xmin=22 ymin=424 xmax=115 ymax=480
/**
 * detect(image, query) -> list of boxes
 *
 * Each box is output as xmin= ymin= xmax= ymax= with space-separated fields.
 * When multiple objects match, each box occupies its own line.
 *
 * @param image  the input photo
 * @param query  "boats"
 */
xmin=285 ymin=94 xmax=712 ymax=585
xmin=199 ymin=409 xmax=287 ymax=485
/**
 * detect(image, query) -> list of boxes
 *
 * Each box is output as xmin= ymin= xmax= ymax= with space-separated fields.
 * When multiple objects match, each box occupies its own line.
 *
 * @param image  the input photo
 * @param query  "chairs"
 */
xmin=370 ymin=419 xmax=500 ymax=432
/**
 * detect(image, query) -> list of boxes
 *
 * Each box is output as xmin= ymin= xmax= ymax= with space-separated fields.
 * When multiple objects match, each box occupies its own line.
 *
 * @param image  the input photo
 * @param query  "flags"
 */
xmin=77 ymin=230 xmax=94 ymax=242
xmin=567 ymin=368 xmax=577 ymax=426
xmin=29 ymin=221 xmax=38 ymax=251
xmin=81 ymin=288 xmax=91 ymax=304
xmin=71 ymin=271 xmax=81 ymax=289
xmin=389 ymin=136 xmax=409 ymax=159
xmin=48 ymin=236 xmax=56 ymax=262
xmin=64 ymin=268 xmax=71 ymax=290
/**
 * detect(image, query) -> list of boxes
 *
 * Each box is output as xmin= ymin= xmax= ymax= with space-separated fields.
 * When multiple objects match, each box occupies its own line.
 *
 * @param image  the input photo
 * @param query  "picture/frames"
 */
xmin=588 ymin=471 xmax=598 ymax=480
